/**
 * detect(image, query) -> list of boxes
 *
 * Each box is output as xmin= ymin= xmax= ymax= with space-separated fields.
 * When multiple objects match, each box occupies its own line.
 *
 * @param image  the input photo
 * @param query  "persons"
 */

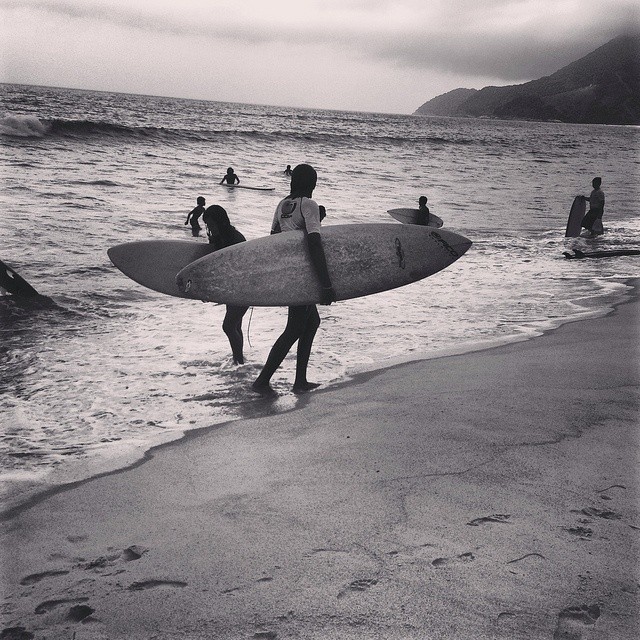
xmin=201 ymin=205 xmax=248 ymax=368
xmin=250 ymin=162 xmax=336 ymax=393
xmin=220 ymin=166 xmax=241 ymax=185
xmin=279 ymin=164 xmax=294 ymax=174
xmin=583 ymin=176 xmax=606 ymax=234
xmin=413 ymin=195 xmax=430 ymax=227
xmin=184 ymin=198 xmax=207 ymax=239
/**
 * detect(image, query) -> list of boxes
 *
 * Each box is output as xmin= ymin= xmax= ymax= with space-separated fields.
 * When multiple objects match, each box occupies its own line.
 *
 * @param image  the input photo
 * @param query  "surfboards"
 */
xmin=107 ymin=241 xmax=214 ymax=298
xmin=175 ymin=225 xmax=472 ymax=306
xmin=215 ymin=183 xmax=275 ymax=190
xmin=566 ymin=195 xmax=586 ymax=237
xmin=388 ymin=208 xmax=443 ymax=227
